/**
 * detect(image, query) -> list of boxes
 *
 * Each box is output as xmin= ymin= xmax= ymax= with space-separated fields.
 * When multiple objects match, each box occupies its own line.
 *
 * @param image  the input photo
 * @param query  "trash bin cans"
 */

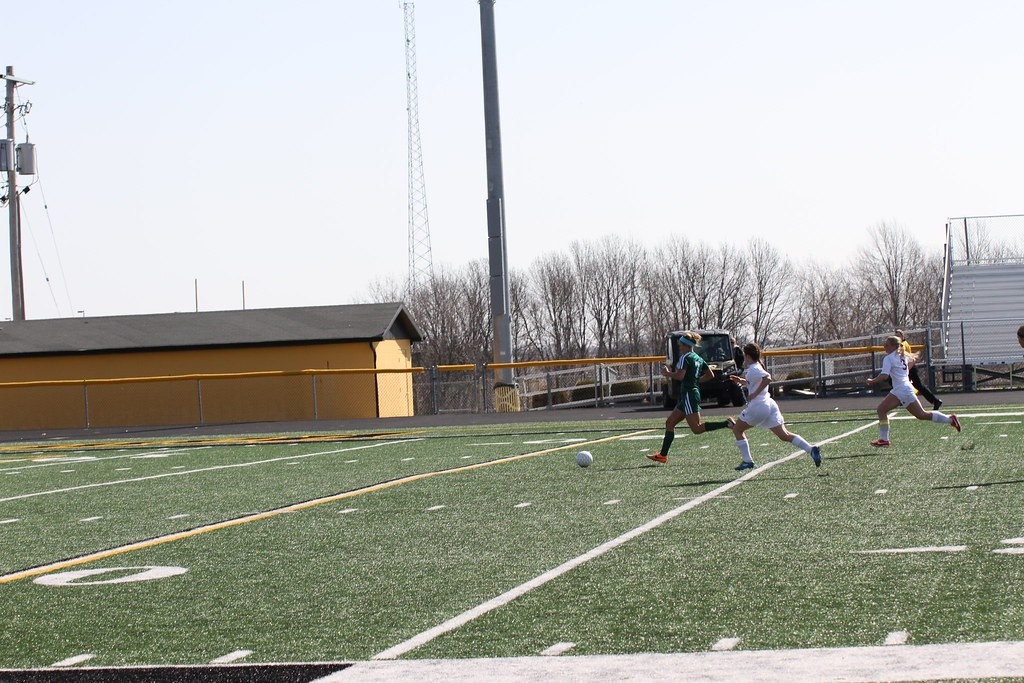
xmin=494 ymin=382 xmax=521 ymax=414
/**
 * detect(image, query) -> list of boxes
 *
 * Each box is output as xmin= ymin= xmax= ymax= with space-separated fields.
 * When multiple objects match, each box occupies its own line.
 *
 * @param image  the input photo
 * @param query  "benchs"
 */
xmin=943 ymin=258 xmax=1024 ymax=363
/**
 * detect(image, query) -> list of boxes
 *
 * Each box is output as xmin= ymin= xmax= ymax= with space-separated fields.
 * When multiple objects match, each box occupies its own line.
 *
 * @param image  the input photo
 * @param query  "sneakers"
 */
xmin=934 ymin=400 xmax=942 ymax=410
xmin=950 ymin=414 xmax=961 ymax=431
xmin=811 ymin=445 xmax=821 ymax=467
xmin=870 ymin=438 xmax=890 ymax=448
xmin=647 ymin=452 xmax=667 ymax=463
xmin=726 ymin=418 xmax=736 ymax=428
xmin=735 ymin=460 xmax=755 ymax=470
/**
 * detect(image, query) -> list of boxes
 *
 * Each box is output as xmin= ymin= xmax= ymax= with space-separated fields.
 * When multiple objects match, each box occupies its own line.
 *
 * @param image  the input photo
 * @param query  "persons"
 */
xmin=865 ymin=335 xmax=962 ymax=447
xmin=727 ymin=343 xmax=821 ymax=472
xmin=646 ymin=331 xmax=744 ymax=463
xmin=886 ymin=328 xmax=943 ymax=413
xmin=1015 ymin=323 xmax=1024 ymax=362
xmin=718 ymin=336 xmax=744 ymax=377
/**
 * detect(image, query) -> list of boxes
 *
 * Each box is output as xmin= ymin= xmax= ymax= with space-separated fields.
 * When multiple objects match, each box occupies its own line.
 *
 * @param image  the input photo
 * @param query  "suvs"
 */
xmin=663 ymin=329 xmax=746 ymax=410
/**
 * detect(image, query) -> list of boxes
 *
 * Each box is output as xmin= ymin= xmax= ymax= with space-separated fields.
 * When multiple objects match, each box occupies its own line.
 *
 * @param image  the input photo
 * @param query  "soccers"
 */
xmin=576 ymin=451 xmax=593 ymax=467
